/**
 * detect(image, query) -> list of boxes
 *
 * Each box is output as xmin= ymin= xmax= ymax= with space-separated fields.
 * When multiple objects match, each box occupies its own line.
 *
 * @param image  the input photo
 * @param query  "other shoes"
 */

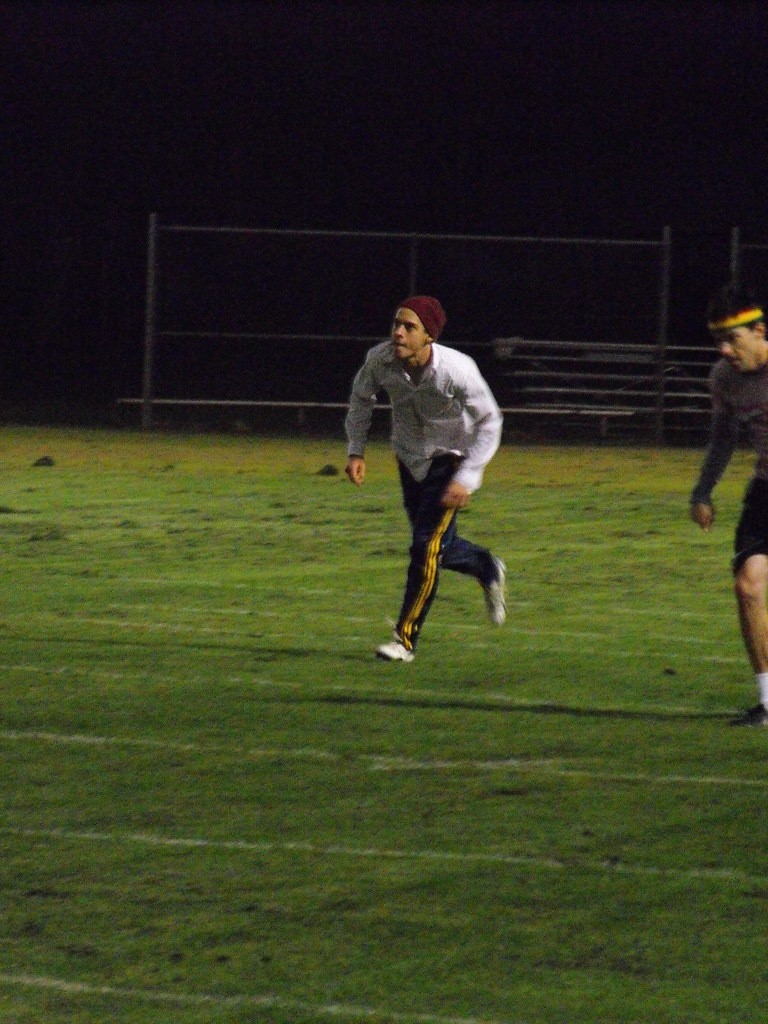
xmin=730 ymin=703 xmax=768 ymax=727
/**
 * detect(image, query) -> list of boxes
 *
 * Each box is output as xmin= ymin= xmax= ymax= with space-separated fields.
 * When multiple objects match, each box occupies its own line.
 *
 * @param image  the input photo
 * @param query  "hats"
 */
xmin=397 ymin=296 xmax=446 ymax=343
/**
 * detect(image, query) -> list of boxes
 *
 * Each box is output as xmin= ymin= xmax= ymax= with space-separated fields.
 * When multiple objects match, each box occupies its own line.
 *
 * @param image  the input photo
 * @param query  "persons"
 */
xmin=690 ymin=297 xmax=768 ymax=725
xmin=345 ymin=296 xmax=506 ymax=662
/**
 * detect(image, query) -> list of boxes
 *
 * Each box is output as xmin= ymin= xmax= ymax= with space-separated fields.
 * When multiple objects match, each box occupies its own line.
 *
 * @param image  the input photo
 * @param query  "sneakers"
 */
xmin=376 ymin=631 xmax=415 ymax=662
xmin=482 ymin=558 xmax=507 ymax=626
